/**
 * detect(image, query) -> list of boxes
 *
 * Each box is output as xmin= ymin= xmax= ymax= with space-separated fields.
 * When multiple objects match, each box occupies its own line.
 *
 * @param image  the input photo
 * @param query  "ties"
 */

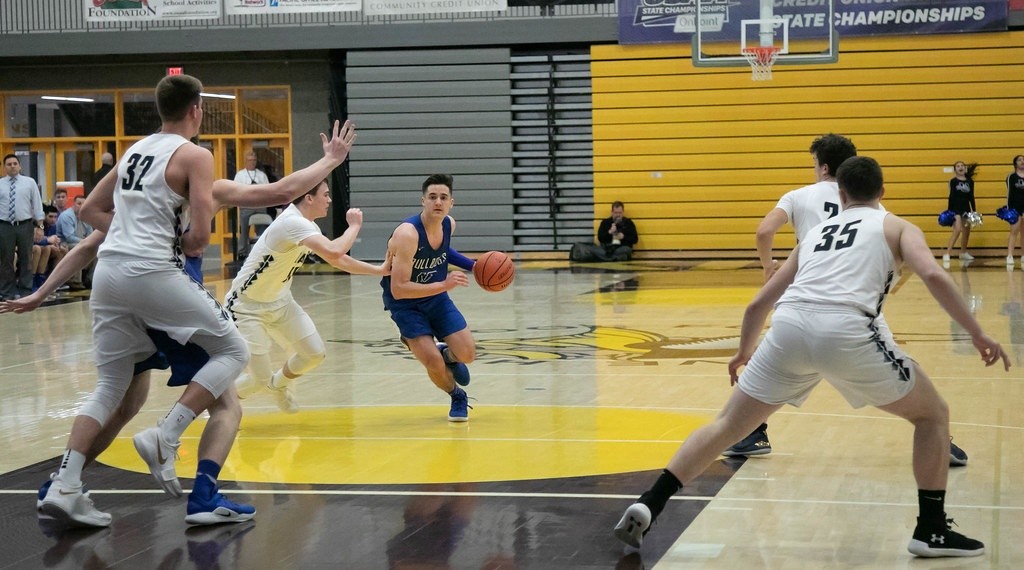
xmin=8 ymin=178 xmax=16 ymax=224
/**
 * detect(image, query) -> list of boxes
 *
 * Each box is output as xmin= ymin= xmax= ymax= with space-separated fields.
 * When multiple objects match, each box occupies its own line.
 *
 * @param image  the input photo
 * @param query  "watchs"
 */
xmin=37 ymin=225 xmax=44 ymax=229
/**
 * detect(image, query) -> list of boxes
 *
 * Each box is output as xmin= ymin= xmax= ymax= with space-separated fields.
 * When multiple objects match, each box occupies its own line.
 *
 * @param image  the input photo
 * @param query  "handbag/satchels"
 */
xmin=569 ymin=242 xmax=599 ymax=262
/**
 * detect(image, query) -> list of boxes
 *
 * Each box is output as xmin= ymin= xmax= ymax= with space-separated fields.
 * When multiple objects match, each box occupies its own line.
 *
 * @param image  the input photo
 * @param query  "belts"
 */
xmin=0 ymin=218 xmax=32 ymax=225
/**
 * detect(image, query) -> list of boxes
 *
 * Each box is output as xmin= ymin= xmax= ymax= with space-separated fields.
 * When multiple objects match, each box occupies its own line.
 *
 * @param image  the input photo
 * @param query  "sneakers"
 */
xmin=613 ymin=503 xmax=652 ymax=549
xmin=436 ymin=342 xmax=470 ymax=387
xmin=42 ymin=473 xmax=112 ymax=526
xmin=949 ymin=435 xmax=968 ymax=464
xmin=132 ymin=427 xmax=182 ymax=499
xmin=722 ymin=422 xmax=772 ymax=455
xmin=268 ymin=374 xmax=299 ymax=414
xmin=943 ymin=254 xmax=950 ymax=261
xmin=959 ymin=252 xmax=974 ymax=259
xmin=1007 ymin=256 xmax=1015 ymax=265
xmin=908 ymin=516 xmax=985 ymax=556
xmin=449 ymin=390 xmax=469 ymax=423
xmin=37 ymin=481 xmax=53 ymax=513
xmin=185 ymin=493 xmax=256 ymax=523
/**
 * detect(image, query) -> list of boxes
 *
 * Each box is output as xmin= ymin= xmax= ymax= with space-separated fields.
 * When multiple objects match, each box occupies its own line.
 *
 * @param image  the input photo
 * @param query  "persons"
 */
xmin=614 ymin=155 xmax=1013 ymax=558
xmin=55 ymin=196 xmax=96 ymax=290
xmin=942 ymin=161 xmax=977 ymax=261
xmin=233 ymin=152 xmax=270 ymax=269
xmin=42 ymin=74 xmax=252 ymax=526
xmin=381 ymin=175 xmax=476 ymax=422
xmin=591 ymin=201 xmax=639 ymax=261
xmin=0 ymin=155 xmax=46 ymax=302
xmin=0 ymin=118 xmax=358 ymax=524
xmin=55 ymin=189 xmax=68 ymax=214
xmin=721 ymin=134 xmax=970 ymax=466
xmin=1005 ymin=155 xmax=1024 ymax=264
xmin=225 ymin=179 xmax=391 ymax=414
xmin=32 ymin=206 xmax=60 ymax=293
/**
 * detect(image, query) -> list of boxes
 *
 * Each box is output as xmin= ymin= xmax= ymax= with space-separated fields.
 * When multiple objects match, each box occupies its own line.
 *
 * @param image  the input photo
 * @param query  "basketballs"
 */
xmin=473 ymin=250 xmax=515 ymax=293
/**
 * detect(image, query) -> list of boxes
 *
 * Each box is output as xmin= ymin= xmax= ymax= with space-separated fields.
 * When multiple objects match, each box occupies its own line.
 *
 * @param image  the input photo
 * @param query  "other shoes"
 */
xmin=73 ymin=282 xmax=86 ymax=290
xmin=60 ymin=284 xmax=70 ymax=290
